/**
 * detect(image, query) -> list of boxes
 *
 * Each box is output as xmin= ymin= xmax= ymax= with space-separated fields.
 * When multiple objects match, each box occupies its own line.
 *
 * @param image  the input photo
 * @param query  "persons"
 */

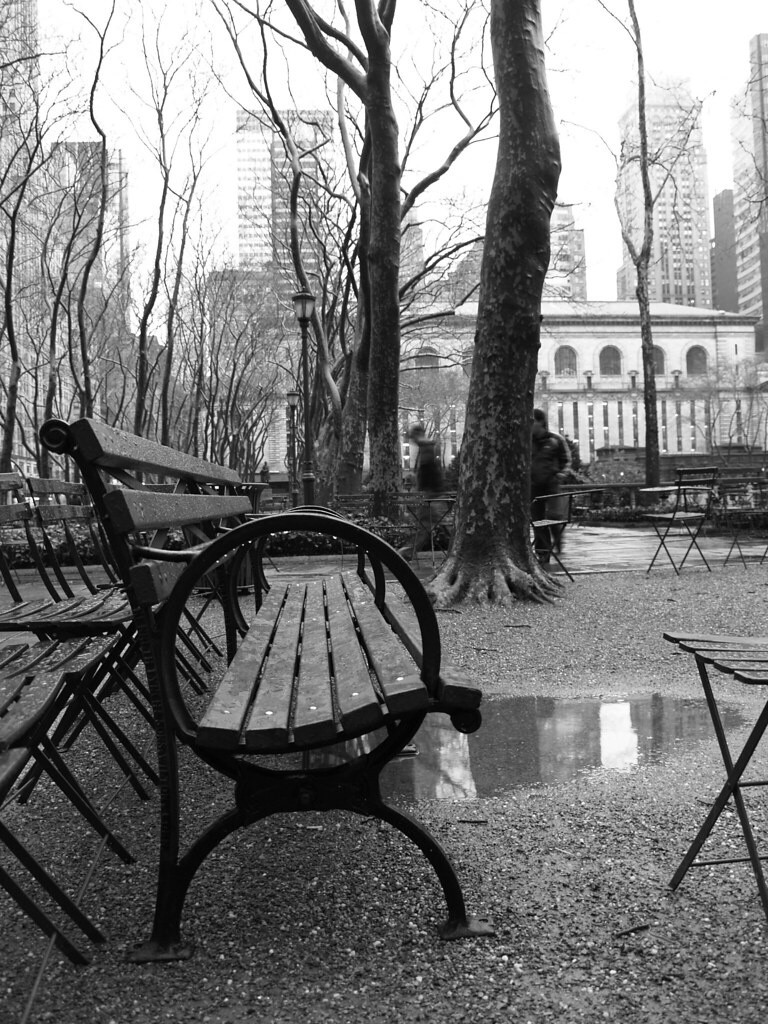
xmin=529 ymin=409 xmax=570 ymax=561
xmin=407 ymin=426 xmax=452 ymax=551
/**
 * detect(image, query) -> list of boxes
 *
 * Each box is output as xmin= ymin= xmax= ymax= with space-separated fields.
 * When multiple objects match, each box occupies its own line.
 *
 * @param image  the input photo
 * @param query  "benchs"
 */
xmin=40 ymin=416 xmax=482 ymax=960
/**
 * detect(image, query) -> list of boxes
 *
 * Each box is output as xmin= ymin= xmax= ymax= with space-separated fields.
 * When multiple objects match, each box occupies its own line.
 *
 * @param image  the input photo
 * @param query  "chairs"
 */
xmin=330 ymin=489 xmax=380 ymax=572
xmin=529 ymin=491 xmax=575 ymax=582
xmin=661 ymin=631 xmax=768 ymax=921
xmin=0 ymin=473 xmax=224 ymax=1024
xmin=638 ymin=466 xmax=768 ymax=577
xmin=369 ymin=490 xmax=456 ymax=568
